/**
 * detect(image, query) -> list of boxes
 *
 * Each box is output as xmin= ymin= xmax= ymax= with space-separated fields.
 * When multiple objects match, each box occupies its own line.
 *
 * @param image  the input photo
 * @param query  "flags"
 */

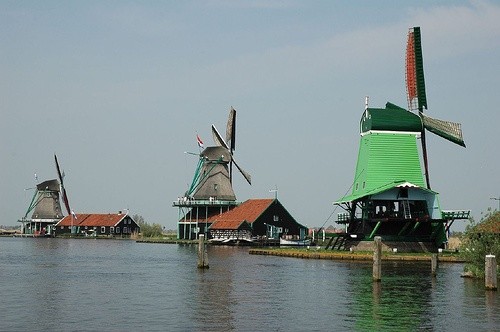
xmin=196 ymin=133 xmax=204 ymax=149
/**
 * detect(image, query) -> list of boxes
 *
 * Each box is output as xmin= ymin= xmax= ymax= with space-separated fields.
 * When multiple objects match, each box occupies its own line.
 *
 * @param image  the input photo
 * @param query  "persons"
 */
xmin=41 ymin=227 xmax=47 ymax=239
xmin=282 ymin=233 xmax=286 ymax=239
xmin=378 ymin=204 xmax=383 ymax=219
xmin=389 ymin=203 xmax=397 ymax=218
